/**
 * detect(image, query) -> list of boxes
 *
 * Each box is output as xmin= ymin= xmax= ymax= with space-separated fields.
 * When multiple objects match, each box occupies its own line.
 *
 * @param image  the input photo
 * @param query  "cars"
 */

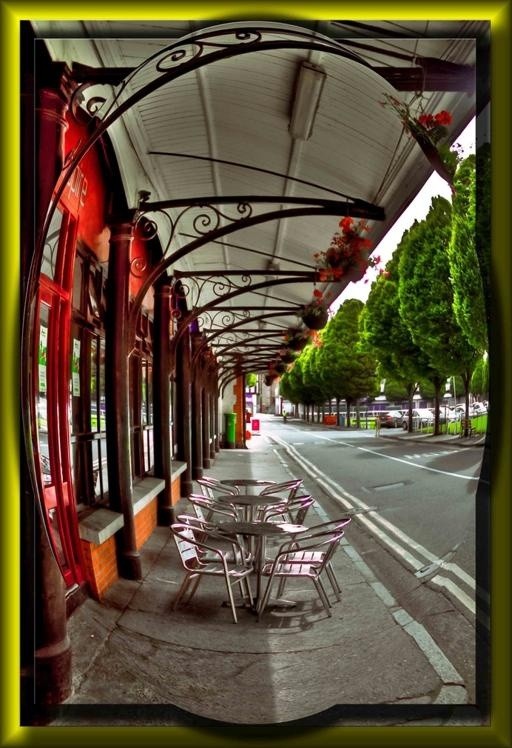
xmin=375 ymin=410 xmax=402 ymax=427
xmin=426 ymin=400 xmax=489 ymax=423
xmin=399 ymin=408 xmax=409 ymax=416
xmin=403 ymin=408 xmax=435 ymax=430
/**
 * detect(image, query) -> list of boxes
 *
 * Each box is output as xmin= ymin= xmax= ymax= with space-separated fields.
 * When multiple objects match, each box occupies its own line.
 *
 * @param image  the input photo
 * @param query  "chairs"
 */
xmin=169 ymin=475 xmax=351 ymax=621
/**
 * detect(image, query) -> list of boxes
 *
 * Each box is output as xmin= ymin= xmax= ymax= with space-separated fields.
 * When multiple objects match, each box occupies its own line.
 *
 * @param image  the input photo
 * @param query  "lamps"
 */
xmin=289 ymin=61 xmax=326 ymax=141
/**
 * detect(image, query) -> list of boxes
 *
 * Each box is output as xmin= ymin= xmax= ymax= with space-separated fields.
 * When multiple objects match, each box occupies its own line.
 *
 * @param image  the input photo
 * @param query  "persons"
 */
xmin=283 ymin=410 xmax=288 ymax=423
xmin=373 ymin=416 xmax=381 ymax=439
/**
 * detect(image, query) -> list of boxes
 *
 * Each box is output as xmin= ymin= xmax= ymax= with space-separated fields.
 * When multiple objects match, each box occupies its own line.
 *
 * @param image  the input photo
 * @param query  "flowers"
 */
xmin=264 ymin=106 xmax=451 ymax=387
